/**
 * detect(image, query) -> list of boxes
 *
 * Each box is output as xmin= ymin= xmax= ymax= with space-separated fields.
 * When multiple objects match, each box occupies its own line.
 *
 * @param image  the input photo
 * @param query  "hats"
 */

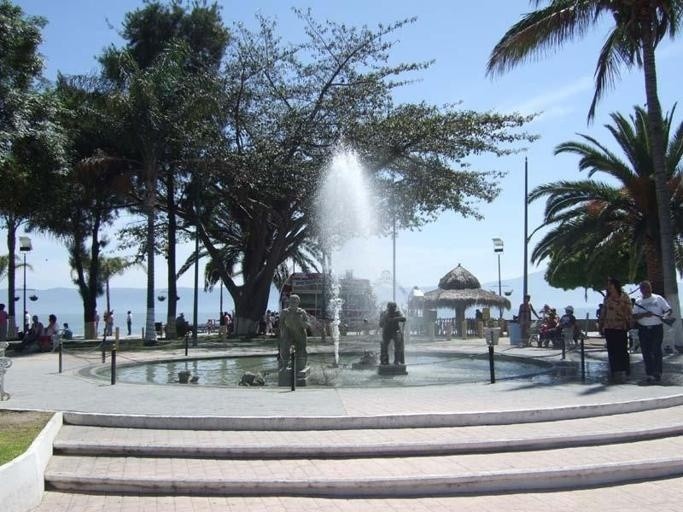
xmin=564 ymin=306 xmax=574 ymax=312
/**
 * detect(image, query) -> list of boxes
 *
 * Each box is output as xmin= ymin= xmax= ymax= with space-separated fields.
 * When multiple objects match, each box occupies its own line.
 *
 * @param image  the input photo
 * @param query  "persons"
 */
xmin=216 ymin=310 xmax=234 ymax=339
xmin=473 ymin=308 xmax=484 ymax=331
xmin=60 ymin=322 xmax=72 ymax=340
xmin=276 ymin=293 xmax=311 ymax=372
xmin=205 ymin=319 xmax=212 ymax=334
xmin=14 ymin=314 xmax=43 ymax=352
xmin=126 ymin=310 xmax=133 ymax=336
xmin=106 ymin=311 xmax=113 ymax=336
xmin=0 ymin=303 xmax=9 ymax=341
xmin=596 ymin=276 xmax=635 ymax=385
xmin=24 ymin=310 xmax=29 ymax=334
xmin=633 ymin=279 xmax=673 ymax=386
xmin=255 ymin=308 xmax=280 ymax=336
xmin=93 ymin=309 xmax=100 ymax=337
xmin=378 ymin=301 xmax=407 ymax=365
xmin=38 ymin=313 xmax=60 ymax=353
xmin=514 ymin=293 xmax=642 ymax=355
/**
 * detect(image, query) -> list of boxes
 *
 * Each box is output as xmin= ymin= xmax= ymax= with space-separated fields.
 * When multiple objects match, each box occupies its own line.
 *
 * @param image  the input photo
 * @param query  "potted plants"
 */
xmin=483 ymin=319 xmax=501 ymax=345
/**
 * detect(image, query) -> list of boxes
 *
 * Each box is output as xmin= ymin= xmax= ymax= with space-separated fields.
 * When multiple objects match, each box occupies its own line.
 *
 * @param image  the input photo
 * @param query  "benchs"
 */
xmin=527 ymin=333 xmax=589 ymax=350
xmin=186 ymin=319 xmax=214 ymax=334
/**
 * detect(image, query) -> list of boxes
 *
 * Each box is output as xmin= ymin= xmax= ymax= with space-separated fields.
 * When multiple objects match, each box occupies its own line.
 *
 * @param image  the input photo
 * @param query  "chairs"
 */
xmin=154 ymin=322 xmax=163 ymax=339
xmin=555 ymin=325 xmax=575 ymax=349
xmin=214 ymin=320 xmax=221 ymax=333
